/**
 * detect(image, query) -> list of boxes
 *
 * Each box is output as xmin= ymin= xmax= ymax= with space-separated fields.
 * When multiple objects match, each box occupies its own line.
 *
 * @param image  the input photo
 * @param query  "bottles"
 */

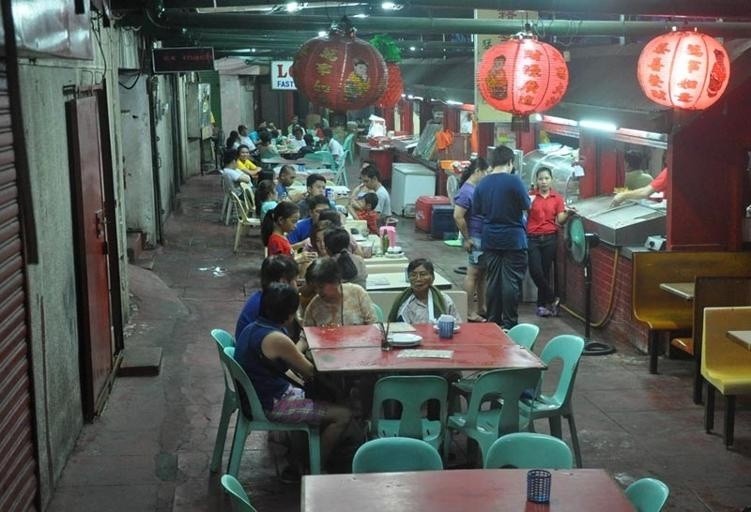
xmin=382 ymin=229 xmax=389 ymax=254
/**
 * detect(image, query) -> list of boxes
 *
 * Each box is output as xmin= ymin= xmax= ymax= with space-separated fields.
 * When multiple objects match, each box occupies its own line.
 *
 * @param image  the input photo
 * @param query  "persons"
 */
xmin=285 ymin=194 xmax=331 ymax=254
xmin=261 ymin=201 xmax=318 ymax=264
xmin=235 ymin=253 xmax=301 ymax=345
xmin=350 ymin=160 xmax=376 ymax=205
xmin=255 ymin=181 xmax=279 ymax=227
xmin=295 ymin=255 xmax=379 ymax=353
xmin=610 ymin=151 xmax=667 ymax=208
xmin=300 ymin=227 xmax=369 ymax=312
xmin=237 ymin=144 xmax=262 ymax=176
xmin=311 ymin=219 xmax=338 ymax=256
xmin=238 ymin=125 xmax=257 ymax=153
xmin=302 ymin=208 xmax=364 ymax=258
xmin=255 ymin=168 xmax=277 ymax=189
xmin=453 ymin=157 xmax=490 ymax=323
xmin=233 ymin=280 xmax=360 ymax=474
xmin=387 ymin=258 xmax=462 ymax=325
xmin=226 ymin=130 xmax=241 ymax=151
xmin=347 ymin=192 xmax=379 ymax=237
xmin=277 ymin=165 xmax=304 ymax=202
xmin=297 ymin=174 xmax=327 ymax=219
xmin=706 ymin=47 xmax=728 ymax=97
xmin=360 ymin=164 xmax=392 ymax=226
xmin=485 ymin=55 xmax=509 ymax=100
xmin=344 ymin=60 xmax=371 ymax=101
xmin=473 ymin=146 xmax=532 ymax=333
xmin=527 ymin=167 xmax=579 ymax=317
xmin=222 ymin=150 xmax=253 ymax=206
xmin=323 ymin=128 xmax=344 ymax=168
xmin=344 ymin=121 xmax=357 ymax=143
xmin=249 ymin=114 xmax=324 ymax=159
xmin=625 ymin=149 xmax=654 ymax=191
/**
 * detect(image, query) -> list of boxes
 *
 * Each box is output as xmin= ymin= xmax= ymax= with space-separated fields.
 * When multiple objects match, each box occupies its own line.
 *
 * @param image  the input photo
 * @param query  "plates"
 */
xmin=386 ymin=252 xmax=404 ymax=257
xmin=388 ymin=333 xmax=423 ymax=347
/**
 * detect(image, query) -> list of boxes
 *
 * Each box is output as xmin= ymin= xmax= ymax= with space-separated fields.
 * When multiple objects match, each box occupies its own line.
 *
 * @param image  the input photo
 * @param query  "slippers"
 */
xmin=466 ymin=316 xmax=487 ymax=323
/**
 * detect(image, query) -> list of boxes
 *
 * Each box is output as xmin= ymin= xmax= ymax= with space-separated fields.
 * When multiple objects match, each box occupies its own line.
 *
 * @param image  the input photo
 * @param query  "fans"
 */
xmin=563 ymin=216 xmax=600 ymax=348
xmin=443 ymin=175 xmax=463 ymax=246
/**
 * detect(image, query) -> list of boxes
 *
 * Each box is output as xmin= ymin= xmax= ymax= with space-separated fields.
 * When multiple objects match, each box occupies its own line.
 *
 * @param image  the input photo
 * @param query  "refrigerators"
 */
xmin=390 ymin=163 xmax=435 ymax=217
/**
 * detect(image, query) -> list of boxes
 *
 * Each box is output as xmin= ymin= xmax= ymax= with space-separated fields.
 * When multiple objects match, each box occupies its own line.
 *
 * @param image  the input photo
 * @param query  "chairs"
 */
xmin=700 ymin=306 xmax=751 ymax=447
xmin=240 ymin=181 xmax=257 ymax=216
xmin=336 ymin=150 xmax=350 ymax=187
xmin=519 ymin=335 xmax=584 ymax=469
xmin=446 ymin=368 xmax=541 ymax=466
xmin=343 ymin=134 xmax=355 ymax=165
xmin=221 ymin=473 xmax=257 ymax=512
xmin=625 ymin=477 xmax=672 ymax=512
xmin=313 ymin=151 xmax=335 ymax=170
xmin=209 ymin=327 xmax=237 ymax=473
xmin=229 ymin=190 xmax=261 ymax=253
xmin=219 ymin=173 xmax=240 ymax=226
xmin=352 ymin=437 xmax=443 ymax=473
xmin=223 ymin=345 xmax=320 ymax=494
xmin=368 ymin=375 xmax=447 ymax=451
xmin=483 ymin=432 xmax=572 ymax=469
xmin=632 ymin=250 xmax=751 ymax=375
xmin=669 ymin=277 xmax=751 ymax=401
xmin=505 ymin=323 xmax=540 ymax=350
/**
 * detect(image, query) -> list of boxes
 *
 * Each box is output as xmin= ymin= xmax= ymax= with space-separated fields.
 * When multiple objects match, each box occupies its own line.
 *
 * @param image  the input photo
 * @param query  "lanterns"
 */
xmin=293 ymin=30 xmax=389 ymax=111
xmin=375 ymin=62 xmax=403 ymax=110
xmin=636 ymin=31 xmax=731 ymax=110
xmin=478 ymin=35 xmax=569 ymax=115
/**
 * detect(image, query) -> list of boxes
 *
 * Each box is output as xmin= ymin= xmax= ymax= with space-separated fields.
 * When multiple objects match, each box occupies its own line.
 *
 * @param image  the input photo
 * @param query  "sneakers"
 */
xmin=551 ymin=298 xmax=560 ymax=316
xmin=502 ymin=325 xmax=512 ymax=332
xmin=279 ymin=467 xmax=302 ymax=484
xmin=536 ymin=306 xmax=550 ymax=317
